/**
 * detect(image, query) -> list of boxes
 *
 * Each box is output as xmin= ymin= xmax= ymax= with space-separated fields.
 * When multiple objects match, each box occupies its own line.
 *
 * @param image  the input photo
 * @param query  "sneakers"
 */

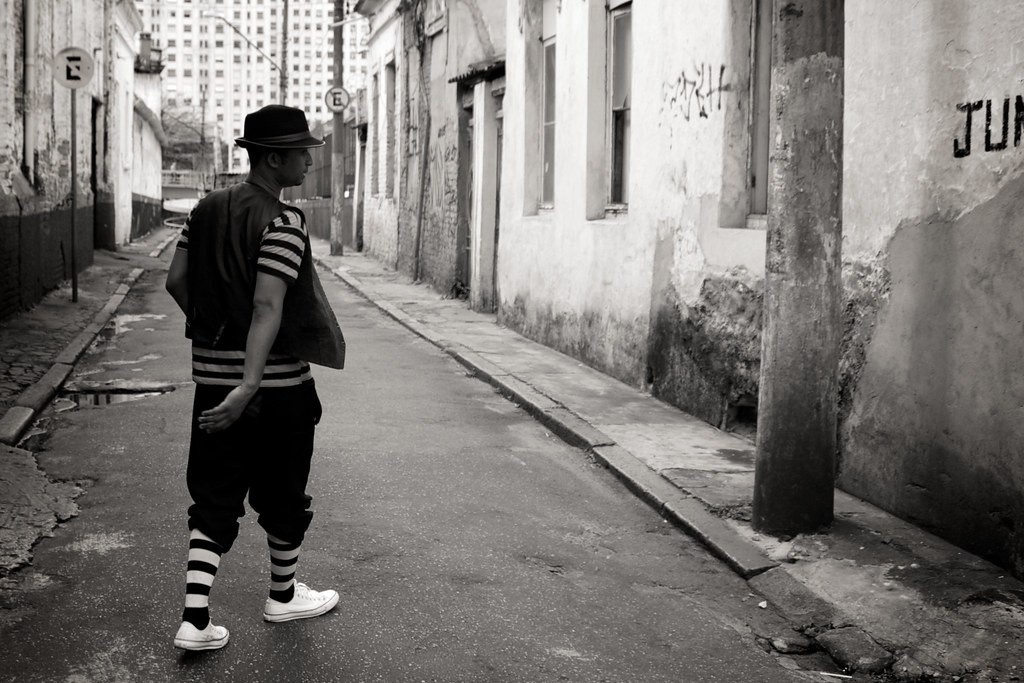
xmin=263 ymin=579 xmax=339 ymax=623
xmin=174 ymin=616 xmax=229 ymax=651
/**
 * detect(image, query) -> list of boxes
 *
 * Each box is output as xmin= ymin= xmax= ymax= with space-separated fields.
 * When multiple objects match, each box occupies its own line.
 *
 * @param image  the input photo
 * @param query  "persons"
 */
xmin=165 ymin=106 xmax=346 ymax=651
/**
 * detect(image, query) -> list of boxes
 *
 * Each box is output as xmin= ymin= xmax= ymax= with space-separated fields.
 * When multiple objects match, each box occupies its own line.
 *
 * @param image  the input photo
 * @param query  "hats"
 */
xmin=234 ymin=105 xmax=326 ymax=148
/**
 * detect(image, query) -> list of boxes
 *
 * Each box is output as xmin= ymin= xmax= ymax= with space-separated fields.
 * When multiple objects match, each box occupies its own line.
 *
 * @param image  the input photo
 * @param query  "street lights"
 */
xmin=203 ymin=9 xmax=290 ymax=106
